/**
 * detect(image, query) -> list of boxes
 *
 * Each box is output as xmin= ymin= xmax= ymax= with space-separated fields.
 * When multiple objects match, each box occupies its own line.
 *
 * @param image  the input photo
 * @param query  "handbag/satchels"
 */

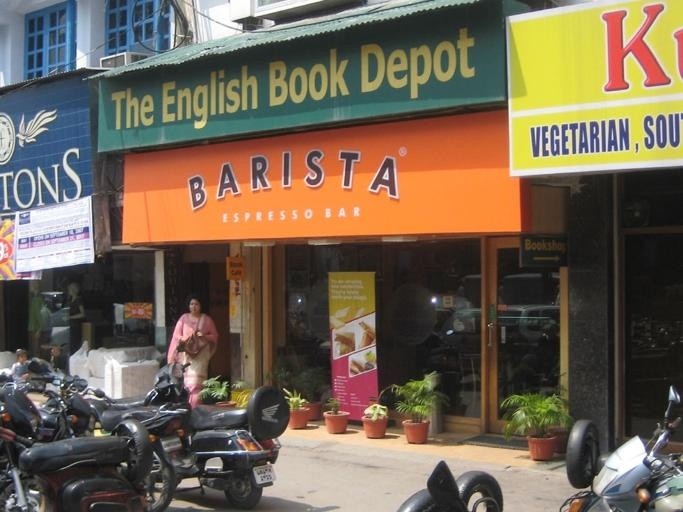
xmin=181 ymin=331 xmax=207 ymax=358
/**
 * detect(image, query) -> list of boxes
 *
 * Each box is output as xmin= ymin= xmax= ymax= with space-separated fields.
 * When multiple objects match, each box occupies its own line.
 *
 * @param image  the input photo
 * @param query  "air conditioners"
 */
xmin=99 ymin=50 xmax=154 ymax=69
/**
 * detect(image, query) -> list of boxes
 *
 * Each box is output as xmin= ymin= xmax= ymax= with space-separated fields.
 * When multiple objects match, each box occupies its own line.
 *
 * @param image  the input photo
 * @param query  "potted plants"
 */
xmin=324 ymin=397 xmax=351 ymax=434
xmin=200 ymin=377 xmax=238 ymax=410
xmin=361 ymin=403 xmax=390 ymax=439
xmin=285 ymin=390 xmax=311 ymax=429
xmin=390 ymin=371 xmax=452 ymax=445
xmin=306 ymin=392 xmax=323 ymax=421
xmin=501 ymin=392 xmax=577 ymax=465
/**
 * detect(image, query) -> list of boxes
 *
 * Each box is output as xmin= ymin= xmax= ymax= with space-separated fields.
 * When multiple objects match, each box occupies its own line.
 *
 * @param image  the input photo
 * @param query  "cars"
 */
xmin=433 ymin=267 xmax=561 ymax=380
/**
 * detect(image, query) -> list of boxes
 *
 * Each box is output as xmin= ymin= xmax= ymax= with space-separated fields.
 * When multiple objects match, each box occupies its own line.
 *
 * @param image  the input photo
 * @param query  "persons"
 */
xmin=165 ymin=297 xmax=219 ymax=409
xmin=11 ymin=349 xmax=32 ymax=377
xmin=62 ymin=282 xmax=85 ymax=354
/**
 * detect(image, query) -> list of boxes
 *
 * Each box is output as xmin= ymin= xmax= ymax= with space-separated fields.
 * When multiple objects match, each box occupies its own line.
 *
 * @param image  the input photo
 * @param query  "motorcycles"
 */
xmin=394 ymin=385 xmax=683 ymax=512
